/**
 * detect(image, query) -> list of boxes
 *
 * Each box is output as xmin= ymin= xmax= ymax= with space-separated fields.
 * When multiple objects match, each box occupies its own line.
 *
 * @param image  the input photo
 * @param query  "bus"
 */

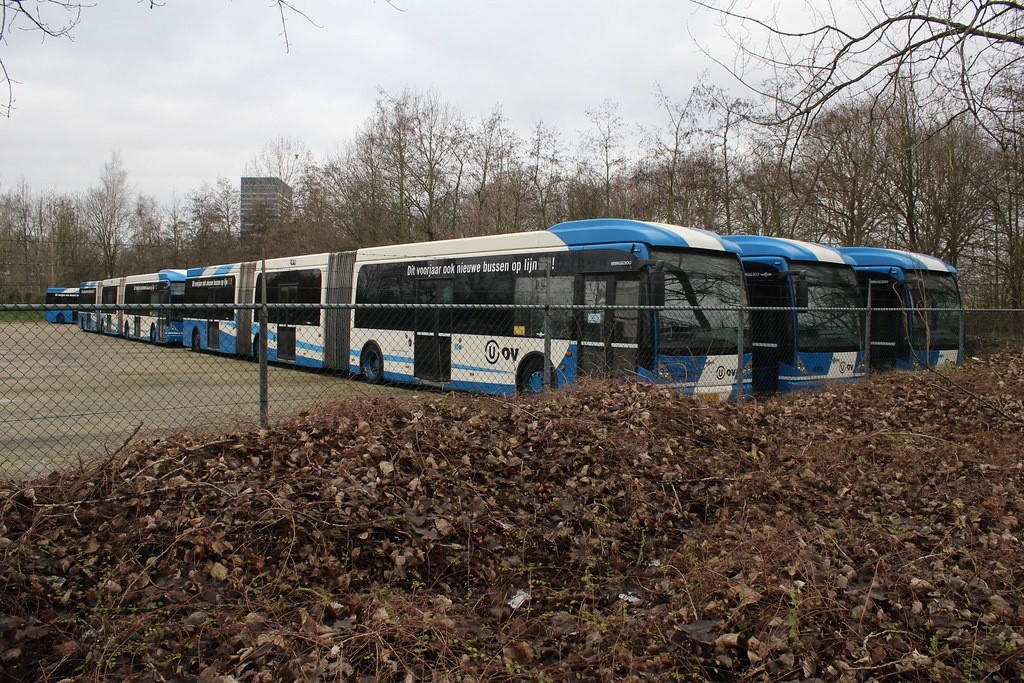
xmin=81 ymin=269 xmax=187 ymax=345
xmin=184 ymin=220 xmax=757 ymax=411
xmin=842 ymin=245 xmax=968 ymax=378
xmin=46 ymin=287 xmax=81 ymax=324
xmin=724 ymin=233 xmax=871 ymax=398
